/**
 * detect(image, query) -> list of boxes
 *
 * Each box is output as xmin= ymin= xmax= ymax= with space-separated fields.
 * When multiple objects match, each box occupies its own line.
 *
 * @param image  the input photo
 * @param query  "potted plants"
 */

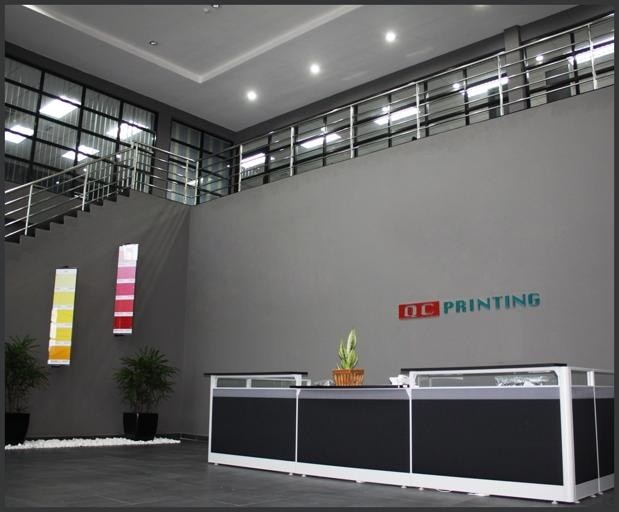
xmin=331 ymin=327 xmax=366 ymax=386
xmin=113 ymin=346 xmax=181 ymax=441
xmin=4 ymin=334 xmax=49 ymax=446
